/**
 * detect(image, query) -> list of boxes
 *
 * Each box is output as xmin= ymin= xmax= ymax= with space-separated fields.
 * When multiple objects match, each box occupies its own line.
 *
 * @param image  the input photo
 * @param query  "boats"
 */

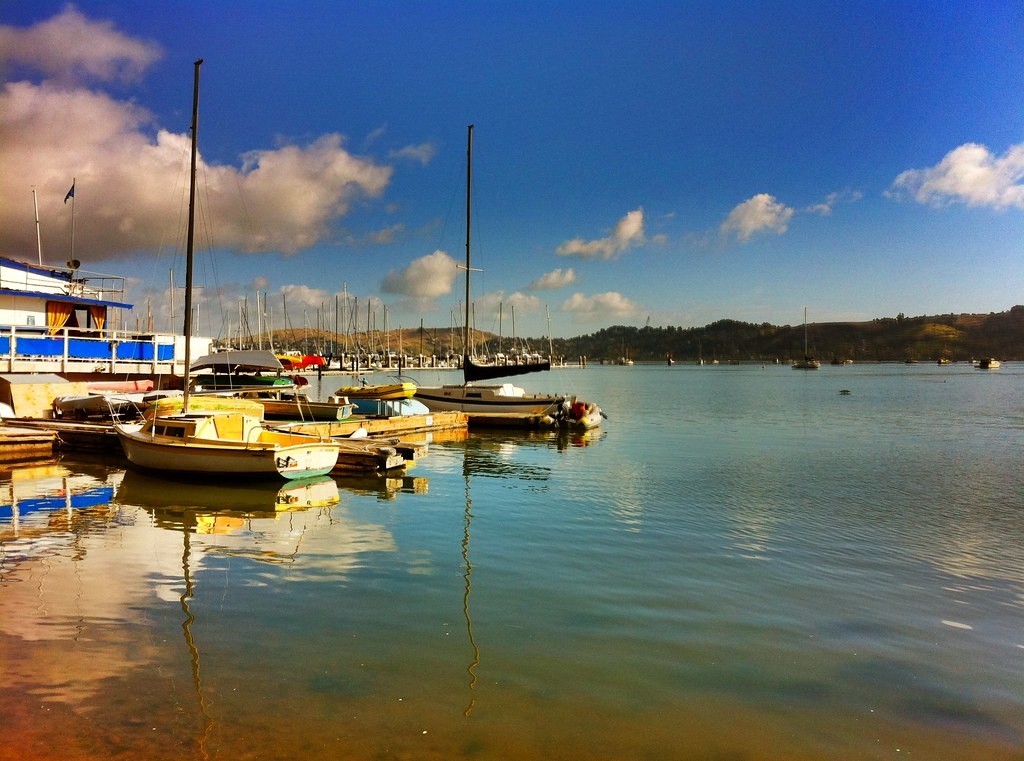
xmin=974 ymin=358 xmax=1001 ymax=369
xmin=539 ymin=399 xmax=609 ymax=431
xmin=937 ymin=358 xmax=951 ymax=364
xmin=188 ymin=349 xmax=311 ymax=396
xmin=335 ymin=382 xmax=417 ymax=400
xmin=831 ymin=358 xmax=853 ymax=365
xmin=791 ymin=356 xmax=820 ymax=369
xmin=463 ymin=348 xmax=551 ymax=383
xmin=113 ymin=394 xmax=342 ymax=481
xmin=905 ymin=358 xmax=918 ymax=364
xmin=0 ymin=256 xmax=215 ymax=462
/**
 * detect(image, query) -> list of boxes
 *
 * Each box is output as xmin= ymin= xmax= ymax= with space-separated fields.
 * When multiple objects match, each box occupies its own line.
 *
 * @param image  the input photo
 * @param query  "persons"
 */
xmin=359 ymin=377 xmax=368 ymax=386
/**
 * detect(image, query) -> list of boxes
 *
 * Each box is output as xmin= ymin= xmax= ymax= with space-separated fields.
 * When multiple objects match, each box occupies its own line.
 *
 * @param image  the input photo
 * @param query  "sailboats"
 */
xmin=414 ymin=129 xmax=579 ymax=423
xmin=620 ymin=337 xmax=791 ymax=366
xmin=216 ymin=288 xmax=524 ymax=367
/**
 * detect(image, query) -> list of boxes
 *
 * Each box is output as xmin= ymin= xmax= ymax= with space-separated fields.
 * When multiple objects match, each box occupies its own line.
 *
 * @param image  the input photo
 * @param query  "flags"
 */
xmin=64 ymin=184 xmax=74 ymax=203
